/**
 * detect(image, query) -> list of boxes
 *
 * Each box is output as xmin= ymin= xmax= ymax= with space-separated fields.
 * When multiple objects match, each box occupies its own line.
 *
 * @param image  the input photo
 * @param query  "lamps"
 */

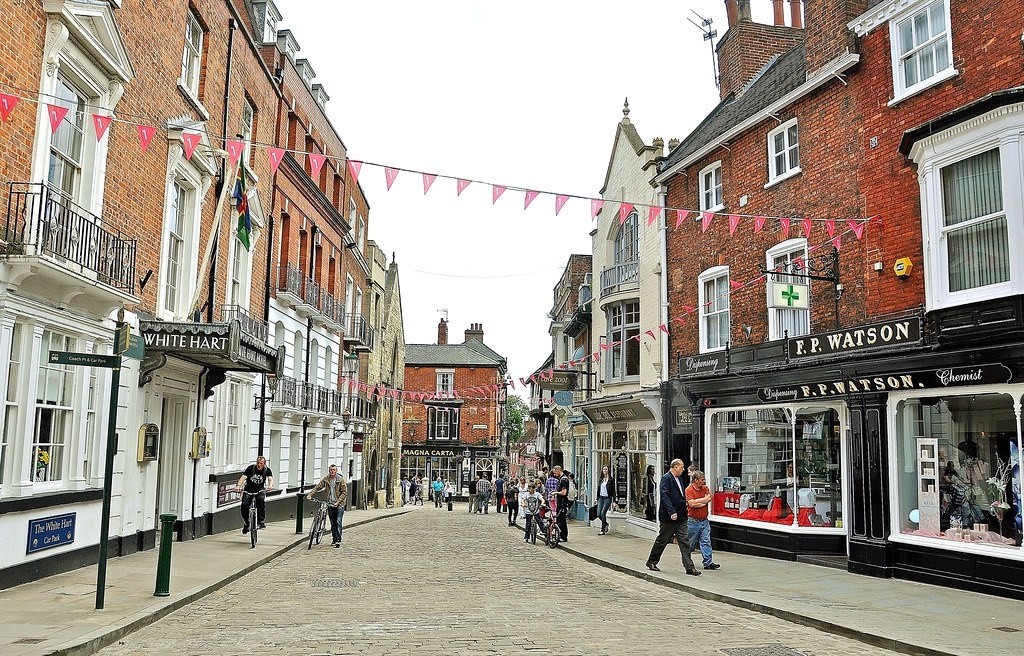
xmin=469 ymin=367 xmax=474 ymax=370
xmin=462 ymin=448 xmax=471 ymax=459
xmin=972 ymin=395 xmax=976 ymax=403
xmin=344 ymin=347 xmax=360 ymax=373
xmin=252 ymin=374 xmax=280 ymax=411
xmin=333 ymin=406 xmax=352 ymax=439
xmin=352 ymin=414 xmax=377 ymax=440
xmin=505 ymin=371 xmax=512 ymax=379
xmin=408 ymin=425 xmax=416 ymax=444
xmin=414 ymin=367 xmax=420 ymax=370
xmin=347 ymin=242 xmax=356 ymax=248
xmin=656 ymin=425 xmax=663 ymax=432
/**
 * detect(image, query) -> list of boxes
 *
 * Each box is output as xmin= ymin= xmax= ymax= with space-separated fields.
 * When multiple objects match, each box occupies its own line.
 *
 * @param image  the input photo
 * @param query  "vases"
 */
xmin=30 ymin=467 xmax=45 ymax=482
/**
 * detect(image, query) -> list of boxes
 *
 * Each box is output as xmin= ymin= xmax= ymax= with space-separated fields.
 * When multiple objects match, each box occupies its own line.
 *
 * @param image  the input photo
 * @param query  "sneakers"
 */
xmin=704 ymin=563 xmax=720 ymax=569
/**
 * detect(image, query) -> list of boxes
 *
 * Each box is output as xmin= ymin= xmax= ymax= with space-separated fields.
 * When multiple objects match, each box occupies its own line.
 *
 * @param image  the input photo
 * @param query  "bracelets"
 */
xmin=236 ymin=485 xmax=240 ymax=487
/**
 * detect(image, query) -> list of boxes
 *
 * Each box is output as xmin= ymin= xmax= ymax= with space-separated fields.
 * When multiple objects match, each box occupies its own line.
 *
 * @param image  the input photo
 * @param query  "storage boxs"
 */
xmin=924 ymin=468 xmax=929 ymax=475
xmin=974 ymin=523 xmax=980 ymax=531
xmin=922 ymin=450 xmax=930 ymax=458
xmin=928 ymin=485 xmax=934 ymax=493
xmin=980 ymin=524 xmax=988 ymax=532
xmin=928 ymin=468 xmax=934 ymax=476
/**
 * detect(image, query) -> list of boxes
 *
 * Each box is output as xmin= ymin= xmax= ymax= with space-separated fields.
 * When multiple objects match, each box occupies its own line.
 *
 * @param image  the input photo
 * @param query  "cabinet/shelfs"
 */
xmin=916 ymin=438 xmax=941 ymax=536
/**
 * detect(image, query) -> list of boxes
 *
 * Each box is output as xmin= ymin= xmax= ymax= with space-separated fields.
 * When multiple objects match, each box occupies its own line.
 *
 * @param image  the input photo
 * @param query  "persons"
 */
xmin=687 ymin=465 xmax=697 ymax=476
xmin=234 ymin=456 xmax=273 ymax=535
xmin=476 ymin=474 xmax=492 ymax=514
xmin=646 ymin=459 xmax=701 ymax=575
xmin=444 ymin=482 xmax=452 ymax=502
xmin=596 ymin=465 xmax=616 ymax=535
xmin=433 ymin=477 xmax=444 ymax=508
xmin=495 ymin=474 xmax=505 ymax=513
xmin=545 ymin=470 xmax=559 ymax=522
xmin=468 ymin=476 xmax=480 ymax=513
xmin=685 ymin=470 xmax=720 ymax=570
xmin=307 ymin=465 xmax=347 ymax=548
xmin=944 ymin=462 xmax=958 ymax=483
xmin=551 ymin=466 xmax=569 ymax=542
xmin=507 ymin=466 xmax=548 ymax=526
xmin=521 ymin=484 xmax=547 ymax=542
xmin=563 ymin=470 xmax=577 ymax=519
xmin=401 ymin=473 xmax=424 ymax=507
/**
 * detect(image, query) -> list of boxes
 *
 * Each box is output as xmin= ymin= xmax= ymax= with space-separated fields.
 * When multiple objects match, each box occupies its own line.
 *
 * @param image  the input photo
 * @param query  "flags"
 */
xmin=234 ymin=150 xmax=252 ymax=253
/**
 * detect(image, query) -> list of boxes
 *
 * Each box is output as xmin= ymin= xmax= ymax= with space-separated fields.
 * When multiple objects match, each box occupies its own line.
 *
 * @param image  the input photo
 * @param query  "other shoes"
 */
xmin=605 ymin=523 xmax=609 ymax=531
xmin=260 ymin=523 xmax=266 ymax=528
xmin=598 ymin=532 xmax=605 ymax=535
xmin=243 ymin=524 xmax=249 ymax=534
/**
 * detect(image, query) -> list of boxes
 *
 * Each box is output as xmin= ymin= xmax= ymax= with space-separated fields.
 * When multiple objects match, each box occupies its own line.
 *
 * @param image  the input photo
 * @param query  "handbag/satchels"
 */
xmin=589 ymin=504 xmax=597 ymax=520
xmin=445 ymin=493 xmax=449 ymax=497
xmin=500 ymin=495 xmax=507 ymax=505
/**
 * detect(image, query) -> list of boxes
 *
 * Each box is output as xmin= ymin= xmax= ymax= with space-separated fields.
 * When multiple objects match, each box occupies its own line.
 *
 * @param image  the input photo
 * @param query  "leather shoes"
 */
xmin=646 ymin=561 xmax=660 ymax=571
xmin=686 ymin=569 xmax=701 ymax=575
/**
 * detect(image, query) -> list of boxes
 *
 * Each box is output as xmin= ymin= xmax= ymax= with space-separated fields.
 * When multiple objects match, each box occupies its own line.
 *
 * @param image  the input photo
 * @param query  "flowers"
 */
xmin=496 ymin=453 xmax=510 ymax=467
xmin=985 ymin=452 xmax=1015 ymax=542
xmin=32 ymin=446 xmax=50 ymax=469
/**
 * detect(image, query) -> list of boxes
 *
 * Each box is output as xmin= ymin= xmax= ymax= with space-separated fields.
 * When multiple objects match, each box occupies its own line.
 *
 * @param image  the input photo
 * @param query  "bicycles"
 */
xmin=236 ymin=487 xmax=268 ymax=548
xmin=306 ymin=497 xmax=337 ymax=550
xmin=540 ymin=505 xmax=567 ymax=549
xmin=521 ymin=504 xmax=545 ymax=545
